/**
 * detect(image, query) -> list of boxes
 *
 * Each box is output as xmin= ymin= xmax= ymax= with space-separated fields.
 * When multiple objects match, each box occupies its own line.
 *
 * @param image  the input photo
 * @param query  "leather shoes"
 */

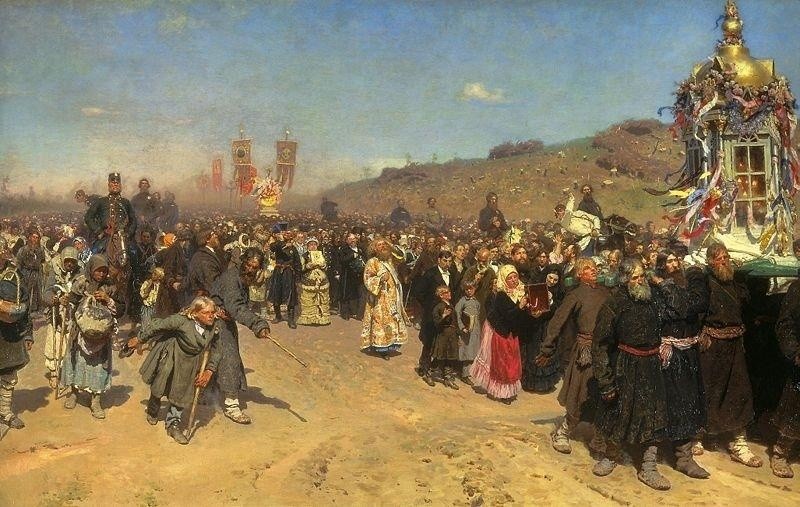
xmin=416 ymin=370 xmax=460 ymax=390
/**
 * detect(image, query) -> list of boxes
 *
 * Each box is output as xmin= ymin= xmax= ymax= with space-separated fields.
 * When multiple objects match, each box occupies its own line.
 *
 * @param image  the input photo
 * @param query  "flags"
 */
xmin=275 ymin=140 xmax=298 ymax=189
xmin=231 ymin=138 xmax=251 ymax=182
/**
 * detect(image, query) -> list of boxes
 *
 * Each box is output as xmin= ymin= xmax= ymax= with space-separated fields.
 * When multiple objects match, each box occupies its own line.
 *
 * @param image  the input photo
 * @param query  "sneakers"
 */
xmin=223 ymin=404 xmax=252 ymax=424
xmin=673 ymin=440 xmax=711 ymax=479
xmin=64 ymin=391 xmax=77 ymax=408
xmin=49 ymin=369 xmax=60 ymax=389
xmin=166 ymin=419 xmax=190 ymax=446
xmin=270 ymin=315 xmax=297 ymax=329
xmin=637 ymin=449 xmax=673 ymax=492
xmin=548 ymin=427 xmax=571 ymax=454
xmin=147 ymin=409 xmax=158 ymax=425
xmin=770 ymin=443 xmax=793 ymax=479
xmin=0 ymin=412 xmax=27 ymax=429
xmin=591 ymin=448 xmax=617 ymax=478
xmin=90 ymin=396 xmax=105 ymax=419
xmin=728 ymin=434 xmax=764 ymax=467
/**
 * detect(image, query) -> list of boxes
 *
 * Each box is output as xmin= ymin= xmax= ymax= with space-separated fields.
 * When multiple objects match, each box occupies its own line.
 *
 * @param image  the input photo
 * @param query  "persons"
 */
xmin=0 ymin=172 xmax=800 ymax=490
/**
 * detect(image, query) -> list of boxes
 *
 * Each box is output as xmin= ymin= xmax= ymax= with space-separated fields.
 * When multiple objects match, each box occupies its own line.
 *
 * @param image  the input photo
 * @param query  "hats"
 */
xmin=108 ymin=172 xmax=121 ymax=181
